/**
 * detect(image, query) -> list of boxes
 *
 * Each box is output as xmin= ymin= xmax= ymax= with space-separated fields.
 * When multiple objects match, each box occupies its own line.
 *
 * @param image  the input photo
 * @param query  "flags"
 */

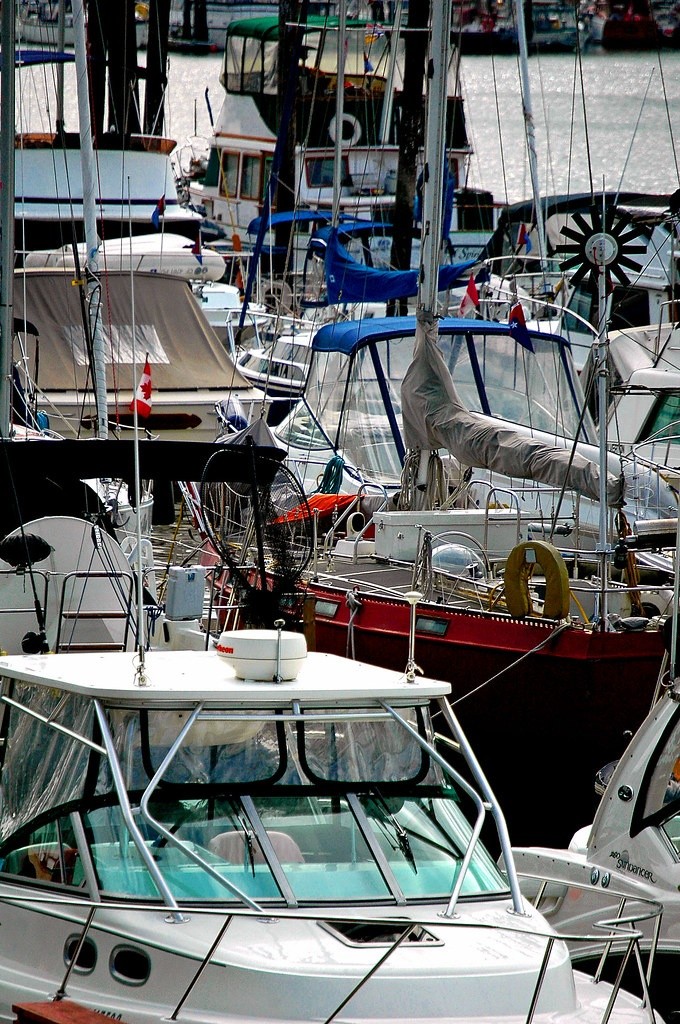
xmin=150 ymin=193 xmax=167 ymax=231
xmin=516 ymin=221 xmax=532 ymax=255
xmin=504 ymin=300 xmax=535 ymax=354
xmin=128 ymin=360 xmax=153 ymax=421
xmin=454 ymin=272 xmax=479 ymax=319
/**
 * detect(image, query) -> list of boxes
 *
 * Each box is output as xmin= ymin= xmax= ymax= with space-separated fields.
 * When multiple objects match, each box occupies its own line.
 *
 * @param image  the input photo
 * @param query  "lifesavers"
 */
xmin=262 ymin=279 xmax=292 ymax=315
xmin=504 ymin=540 xmax=570 ymax=621
xmin=329 ymin=113 xmax=362 ymax=146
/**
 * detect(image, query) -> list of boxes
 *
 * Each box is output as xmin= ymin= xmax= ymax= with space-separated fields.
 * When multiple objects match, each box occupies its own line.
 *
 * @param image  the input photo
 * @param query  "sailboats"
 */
xmin=0 ymin=0 xmax=680 ymax=1024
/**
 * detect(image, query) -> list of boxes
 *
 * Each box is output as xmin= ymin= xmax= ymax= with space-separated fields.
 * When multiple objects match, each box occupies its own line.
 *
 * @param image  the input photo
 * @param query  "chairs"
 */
xmin=206 ymin=829 xmax=306 ymax=866
